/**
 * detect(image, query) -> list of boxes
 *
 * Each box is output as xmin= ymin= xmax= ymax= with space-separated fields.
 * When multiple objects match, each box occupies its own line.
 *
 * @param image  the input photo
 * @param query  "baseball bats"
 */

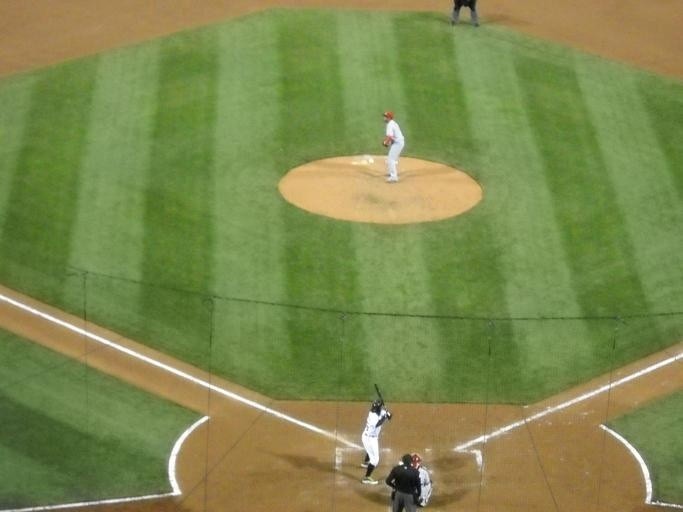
xmin=374 ymin=384 xmax=391 ymax=420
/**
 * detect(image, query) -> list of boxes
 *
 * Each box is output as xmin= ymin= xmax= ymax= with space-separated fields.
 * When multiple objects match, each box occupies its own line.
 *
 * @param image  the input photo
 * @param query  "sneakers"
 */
xmin=361 ymin=462 xmax=368 ymax=467
xmin=361 ymin=476 xmax=378 ymax=484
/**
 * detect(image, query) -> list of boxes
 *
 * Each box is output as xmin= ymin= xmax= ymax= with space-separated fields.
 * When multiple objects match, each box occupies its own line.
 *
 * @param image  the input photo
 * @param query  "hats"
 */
xmin=383 ymin=111 xmax=393 ymax=119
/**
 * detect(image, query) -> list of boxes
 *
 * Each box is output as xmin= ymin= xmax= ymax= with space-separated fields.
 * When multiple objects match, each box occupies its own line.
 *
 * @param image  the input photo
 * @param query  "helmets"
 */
xmin=373 ymin=399 xmax=381 ymax=408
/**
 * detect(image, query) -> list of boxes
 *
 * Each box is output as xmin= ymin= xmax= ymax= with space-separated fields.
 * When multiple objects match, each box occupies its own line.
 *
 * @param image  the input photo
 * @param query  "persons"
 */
xmin=386 ymin=453 xmax=433 ymax=512
xmin=382 ymin=111 xmax=404 ymax=183
xmin=449 ymin=0 xmax=480 ymax=26
xmin=361 ymin=399 xmax=392 ymax=485
